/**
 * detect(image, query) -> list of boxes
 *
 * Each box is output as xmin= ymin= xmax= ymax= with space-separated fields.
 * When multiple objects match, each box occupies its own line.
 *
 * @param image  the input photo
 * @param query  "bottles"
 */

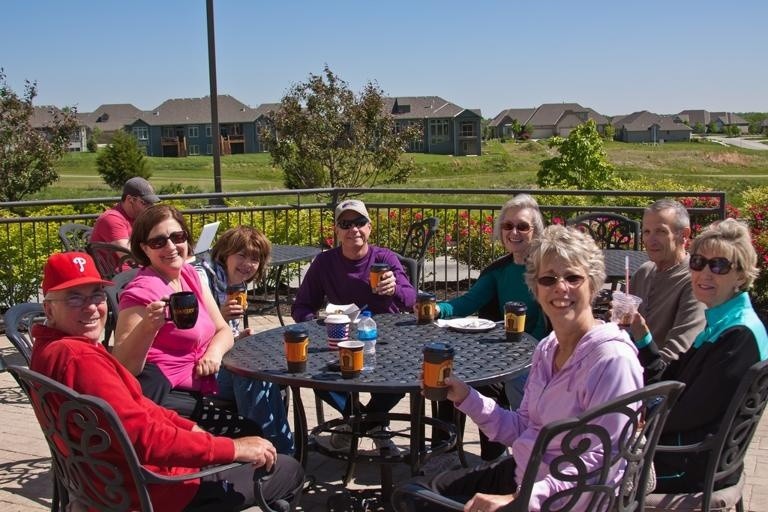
xmin=357 ymin=311 xmax=378 ymax=371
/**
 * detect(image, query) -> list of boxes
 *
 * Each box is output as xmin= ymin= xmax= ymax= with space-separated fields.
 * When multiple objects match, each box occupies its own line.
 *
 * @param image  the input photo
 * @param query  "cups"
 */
xmin=159 ymin=291 xmax=199 ymax=329
xmin=415 ymin=292 xmax=436 ymax=323
xmin=503 ymin=300 xmax=528 ymax=342
xmin=423 ymin=341 xmax=454 ymax=401
xmin=337 ymin=341 xmax=364 ymax=379
xmin=610 ymin=290 xmax=644 ymax=331
xmin=284 ymin=325 xmax=310 ymax=373
xmin=369 ymin=264 xmax=390 ymax=295
xmin=226 ymin=284 xmax=248 ymax=317
xmin=324 ymin=314 xmax=351 ymax=351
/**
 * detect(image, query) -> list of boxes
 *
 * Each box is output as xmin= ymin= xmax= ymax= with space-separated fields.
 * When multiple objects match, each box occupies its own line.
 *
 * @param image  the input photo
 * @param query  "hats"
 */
xmin=123 ymin=178 xmax=162 ymax=203
xmin=43 ymin=251 xmax=116 ymax=294
xmin=334 ymin=200 xmax=370 ymax=223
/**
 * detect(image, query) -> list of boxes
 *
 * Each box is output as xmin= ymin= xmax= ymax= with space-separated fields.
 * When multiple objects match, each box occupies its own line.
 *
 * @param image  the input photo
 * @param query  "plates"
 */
xmin=446 ymin=318 xmax=496 ymax=333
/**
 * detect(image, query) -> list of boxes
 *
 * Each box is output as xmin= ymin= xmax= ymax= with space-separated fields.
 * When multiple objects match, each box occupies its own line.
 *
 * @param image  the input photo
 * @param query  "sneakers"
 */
xmin=419 ymin=451 xmax=462 ymax=476
xmin=330 ymin=414 xmax=364 ymax=449
xmin=367 ymin=424 xmax=404 ymax=457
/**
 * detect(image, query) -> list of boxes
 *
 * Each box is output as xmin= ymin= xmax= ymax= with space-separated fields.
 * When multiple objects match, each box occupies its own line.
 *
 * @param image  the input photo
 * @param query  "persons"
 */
xmin=423 ymin=224 xmax=645 ymax=510
xmin=291 ymin=197 xmax=416 ymax=448
xmin=28 ymin=176 xmax=303 ymax=511
xmin=418 ymin=195 xmax=553 ymax=461
xmin=608 ymin=196 xmax=709 ymax=415
xmin=643 ymin=216 xmax=768 ymax=488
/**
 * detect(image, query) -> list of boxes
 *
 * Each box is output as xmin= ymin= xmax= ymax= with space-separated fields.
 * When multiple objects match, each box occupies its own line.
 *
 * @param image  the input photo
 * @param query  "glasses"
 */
xmin=689 ymin=254 xmax=741 ymax=276
xmin=43 ymin=290 xmax=107 ymax=308
xmin=144 ymin=229 xmax=189 ymax=249
xmin=338 ymin=216 xmax=368 ymax=230
xmin=534 ymin=272 xmax=592 ymax=290
xmin=500 ymin=221 xmax=534 ymax=234
xmin=130 ymin=194 xmax=154 ymax=206
